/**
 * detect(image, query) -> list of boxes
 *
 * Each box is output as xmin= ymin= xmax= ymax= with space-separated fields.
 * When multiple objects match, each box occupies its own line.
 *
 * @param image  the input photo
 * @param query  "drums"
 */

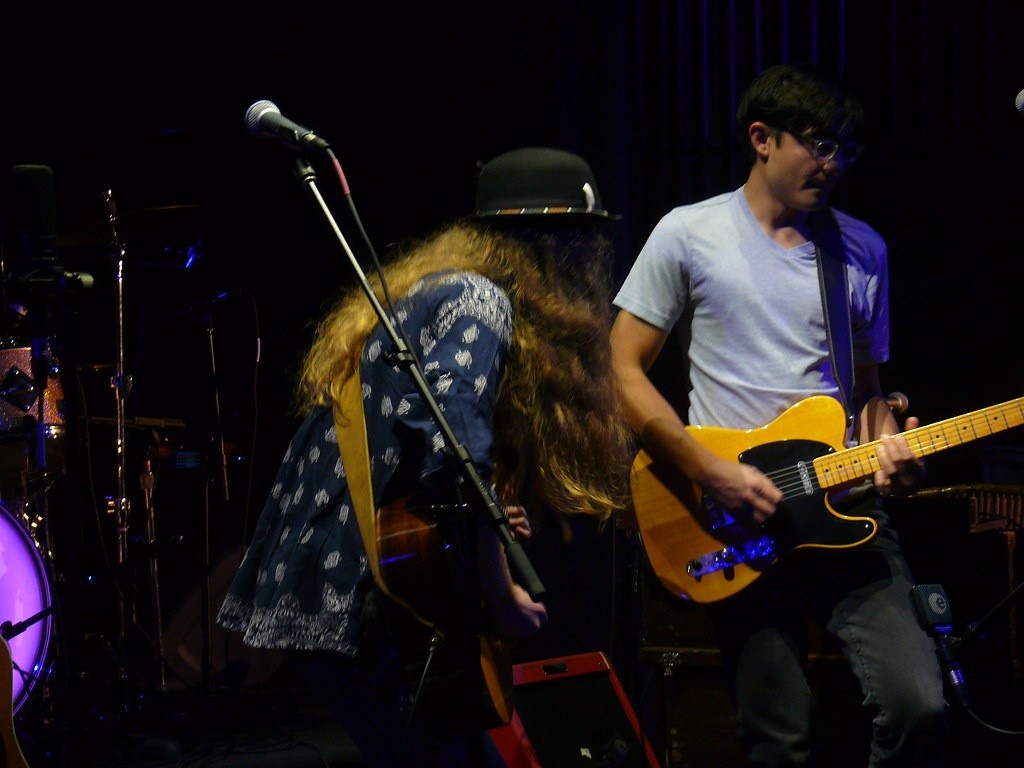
xmin=0 ymin=501 xmax=59 ymax=718
xmin=72 ymin=203 xmax=232 ymax=333
xmin=0 ymin=332 xmax=67 ymax=498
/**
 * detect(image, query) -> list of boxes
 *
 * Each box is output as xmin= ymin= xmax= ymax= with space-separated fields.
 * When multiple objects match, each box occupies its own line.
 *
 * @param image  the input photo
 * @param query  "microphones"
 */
xmin=245 ymin=100 xmax=330 ymax=151
xmin=1015 ymin=89 xmax=1024 ymax=115
xmin=49 ymin=267 xmax=95 ymax=290
xmin=168 ymin=287 xmax=244 ymax=322
xmin=911 ymin=584 xmax=969 ymax=710
xmin=10 ymin=164 xmax=58 ymax=283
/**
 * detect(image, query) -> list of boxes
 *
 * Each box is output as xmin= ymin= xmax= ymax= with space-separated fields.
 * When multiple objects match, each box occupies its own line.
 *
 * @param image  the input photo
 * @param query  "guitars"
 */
xmin=627 ymin=394 xmax=1024 ymax=603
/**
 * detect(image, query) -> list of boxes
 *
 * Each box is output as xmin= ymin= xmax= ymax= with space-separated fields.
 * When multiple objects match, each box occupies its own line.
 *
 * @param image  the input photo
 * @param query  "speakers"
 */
xmin=487 ymin=652 xmax=662 ymax=768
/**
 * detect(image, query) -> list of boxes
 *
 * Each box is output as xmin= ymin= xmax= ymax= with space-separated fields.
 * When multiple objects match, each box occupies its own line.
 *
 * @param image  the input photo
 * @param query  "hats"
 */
xmin=455 ymin=147 xmax=623 ymax=230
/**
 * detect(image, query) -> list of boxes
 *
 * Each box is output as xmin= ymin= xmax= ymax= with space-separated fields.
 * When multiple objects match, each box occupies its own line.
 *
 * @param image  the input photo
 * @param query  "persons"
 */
xmin=215 ymin=148 xmax=632 ymax=767
xmin=608 ymin=65 xmax=949 ymax=768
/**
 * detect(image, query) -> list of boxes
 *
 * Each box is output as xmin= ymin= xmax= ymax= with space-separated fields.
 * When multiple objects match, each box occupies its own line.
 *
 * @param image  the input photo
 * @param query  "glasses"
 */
xmin=772 ymin=121 xmax=864 ymax=165
